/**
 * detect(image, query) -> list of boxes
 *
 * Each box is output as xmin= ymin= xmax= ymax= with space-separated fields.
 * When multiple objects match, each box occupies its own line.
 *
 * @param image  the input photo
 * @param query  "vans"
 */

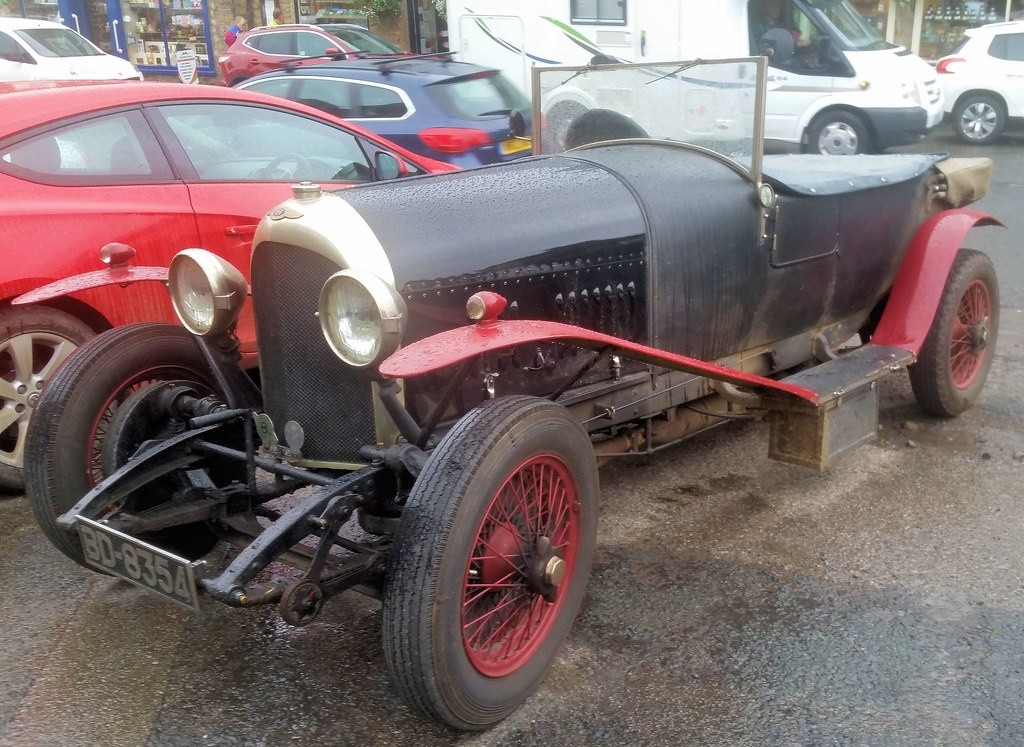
xmin=446 ymin=0 xmax=944 ymax=159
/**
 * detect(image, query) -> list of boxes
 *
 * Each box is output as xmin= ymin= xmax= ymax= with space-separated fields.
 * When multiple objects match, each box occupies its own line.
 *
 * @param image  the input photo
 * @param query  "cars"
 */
xmin=0 ymin=82 xmax=465 ymax=493
xmin=207 ymin=59 xmax=548 ymax=174
xmin=19 ymin=54 xmax=1009 ymax=738
xmin=1 ymin=16 xmax=146 ymax=83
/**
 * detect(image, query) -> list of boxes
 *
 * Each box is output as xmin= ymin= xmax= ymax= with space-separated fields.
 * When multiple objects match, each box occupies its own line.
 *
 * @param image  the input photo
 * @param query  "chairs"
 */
xmin=110 ymin=135 xmax=137 ymax=173
xmin=11 ymin=137 xmax=62 ymax=173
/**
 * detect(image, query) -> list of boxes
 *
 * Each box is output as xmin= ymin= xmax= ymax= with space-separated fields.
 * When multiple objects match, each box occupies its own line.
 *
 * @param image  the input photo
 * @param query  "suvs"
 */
xmin=934 ymin=21 xmax=1024 ymax=145
xmin=219 ymin=23 xmax=443 ymax=89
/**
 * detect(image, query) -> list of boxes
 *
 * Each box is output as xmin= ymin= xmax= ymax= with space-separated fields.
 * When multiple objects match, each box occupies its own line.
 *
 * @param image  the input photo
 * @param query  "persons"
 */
xmin=269 ymin=7 xmax=285 ymax=27
xmin=751 ymin=0 xmax=810 ymax=48
xmin=225 ymin=15 xmax=246 ymax=47
